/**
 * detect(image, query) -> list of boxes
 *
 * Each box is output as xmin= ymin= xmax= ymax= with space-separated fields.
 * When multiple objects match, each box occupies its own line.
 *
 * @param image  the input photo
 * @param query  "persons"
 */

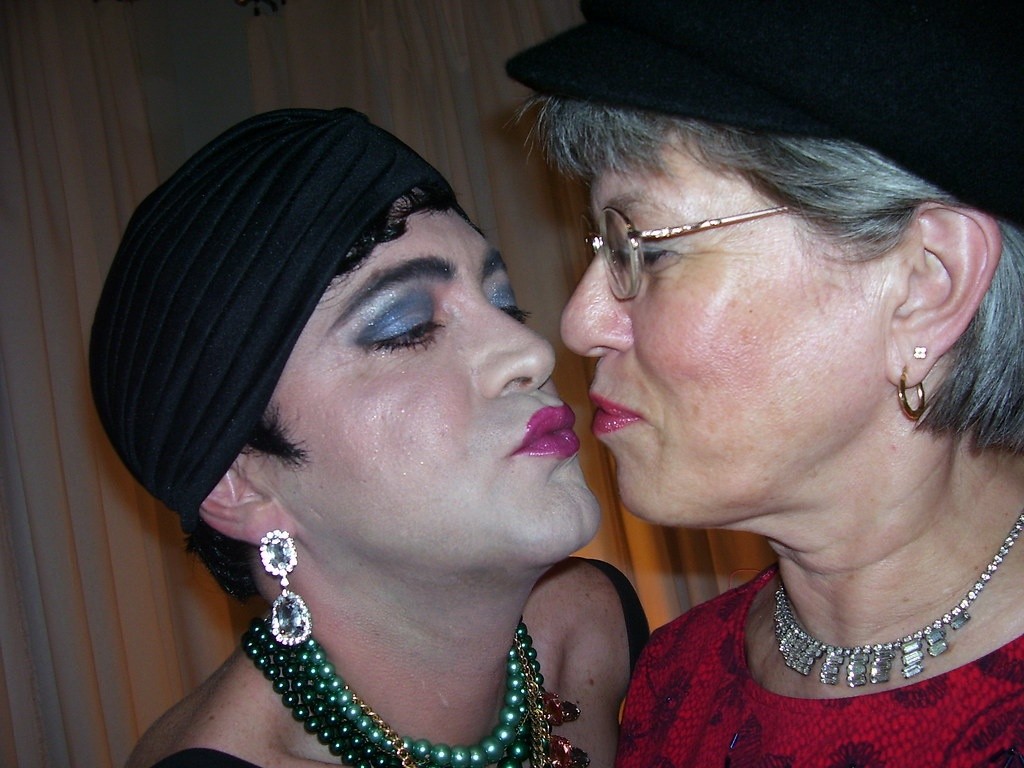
xmin=87 ymin=104 xmax=650 ymax=768
xmin=505 ymin=0 xmax=1024 ymax=768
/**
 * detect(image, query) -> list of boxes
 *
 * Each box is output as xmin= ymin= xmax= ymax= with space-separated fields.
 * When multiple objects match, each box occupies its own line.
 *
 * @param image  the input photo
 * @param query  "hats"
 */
xmin=503 ymin=1 xmax=1024 ymax=228
xmin=88 ymin=107 xmax=451 ymax=517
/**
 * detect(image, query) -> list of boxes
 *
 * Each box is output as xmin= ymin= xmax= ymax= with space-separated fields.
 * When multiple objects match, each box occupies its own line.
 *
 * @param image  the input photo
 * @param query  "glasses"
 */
xmin=578 ymin=207 xmax=790 ymax=297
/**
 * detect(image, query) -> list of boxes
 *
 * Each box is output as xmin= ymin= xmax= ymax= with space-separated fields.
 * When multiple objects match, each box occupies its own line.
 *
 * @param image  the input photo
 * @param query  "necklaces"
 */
xmin=771 ymin=518 xmax=1023 ymax=688
xmin=244 ymin=599 xmax=591 ymax=768
